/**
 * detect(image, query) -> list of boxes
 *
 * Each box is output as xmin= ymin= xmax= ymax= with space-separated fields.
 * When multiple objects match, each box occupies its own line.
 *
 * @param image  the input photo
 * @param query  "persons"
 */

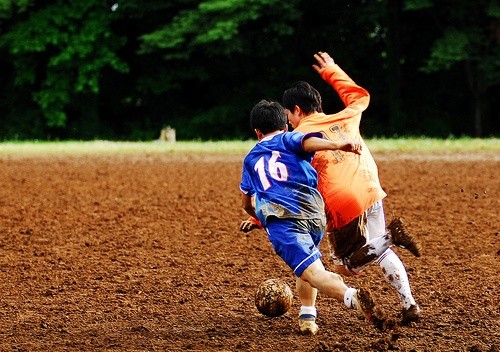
xmin=241 ymin=100 xmax=389 ymax=336
xmin=238 ymin=52 xmax=426 ymax=324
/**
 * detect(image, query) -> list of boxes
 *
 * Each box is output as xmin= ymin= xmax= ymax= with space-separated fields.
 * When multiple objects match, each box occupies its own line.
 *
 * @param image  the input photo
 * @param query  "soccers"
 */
xmin=255 ymin=279 xmax=292 ymax=318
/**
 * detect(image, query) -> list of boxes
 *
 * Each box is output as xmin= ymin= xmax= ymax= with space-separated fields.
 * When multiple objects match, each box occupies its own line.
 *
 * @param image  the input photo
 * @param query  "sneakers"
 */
xmin=356 ymin=287 xmax=390 ymax=332
xmin=397 ymin=305 xmax=421 ymax=326
xmin=387 ymin=218 xmax=421 ymax=257
xmin=298 ymin=314 xmax=320 ymax=335
